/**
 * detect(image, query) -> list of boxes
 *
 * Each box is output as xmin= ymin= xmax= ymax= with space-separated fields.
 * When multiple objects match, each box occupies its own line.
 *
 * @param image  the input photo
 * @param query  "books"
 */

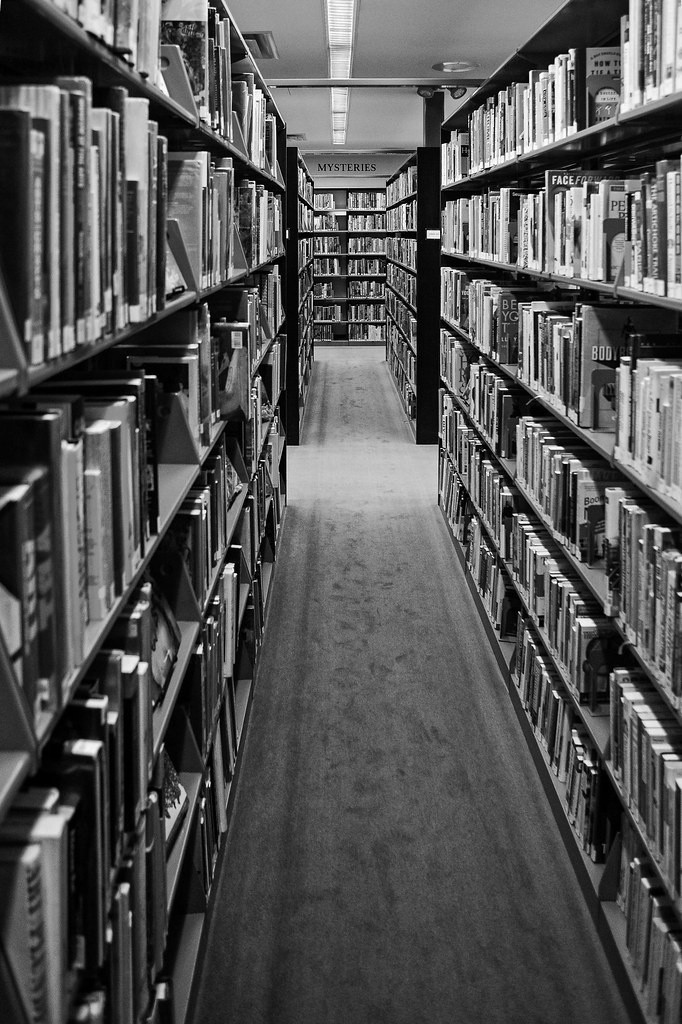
xmin=313 ymin=187 xmax=387 ymax=343
xmin=0 ymin=0 xmax=313 ymax=1023
xmin=385 ymin=0 xmax=682 ymax=1022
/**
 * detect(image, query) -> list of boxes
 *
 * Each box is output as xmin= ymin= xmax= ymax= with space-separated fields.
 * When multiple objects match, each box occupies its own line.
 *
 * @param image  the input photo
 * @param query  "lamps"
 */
xmin=415 ymin=84 xmax=439 ymax=98
xmin=446 ymin=85 xmax=467 ymax=99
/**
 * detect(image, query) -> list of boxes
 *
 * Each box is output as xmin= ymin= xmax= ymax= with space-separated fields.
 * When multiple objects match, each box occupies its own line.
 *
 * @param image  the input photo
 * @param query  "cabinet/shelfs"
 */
xmin=0 ymin=0 xmax=682 ymax=1024
